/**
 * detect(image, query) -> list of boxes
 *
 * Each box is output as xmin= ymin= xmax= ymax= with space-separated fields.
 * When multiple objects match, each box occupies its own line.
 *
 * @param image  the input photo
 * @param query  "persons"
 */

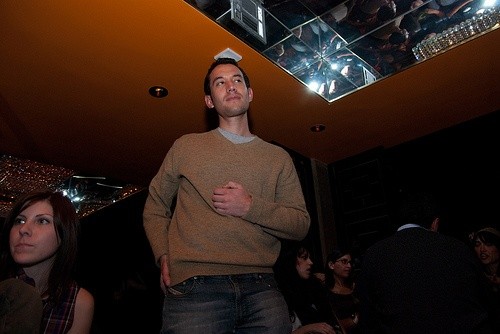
xmin=270 ymin=0 xmax=500 ymax=105
xmin=0 ymin=191 xmax=94 ymax=334
xmin=142 ymin=58 xmax=311 ymax=334
xmin=321 ymin=247 xmax=361 ymax=333
xmin=473 ymin=226 xmax=500 ymax=294
xmin=358 ymin=195 xmax=500 ymax=333
xmin=275 ymin=239 xmax=336 ymax=334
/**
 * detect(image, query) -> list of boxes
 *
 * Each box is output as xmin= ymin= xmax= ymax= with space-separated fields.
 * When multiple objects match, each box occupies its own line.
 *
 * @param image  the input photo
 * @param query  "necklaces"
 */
xmin=484 ymin=269 xmax=498 ymax=282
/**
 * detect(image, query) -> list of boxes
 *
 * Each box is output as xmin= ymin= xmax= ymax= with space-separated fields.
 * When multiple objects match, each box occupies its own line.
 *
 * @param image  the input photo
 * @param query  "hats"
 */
xmin=473 ymin=227 xmax=500 ymax=237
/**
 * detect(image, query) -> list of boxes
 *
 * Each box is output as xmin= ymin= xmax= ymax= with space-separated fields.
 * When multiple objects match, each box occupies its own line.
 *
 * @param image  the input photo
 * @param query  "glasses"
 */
xmin=335 ymin=258 xmax=353 ymax=265
